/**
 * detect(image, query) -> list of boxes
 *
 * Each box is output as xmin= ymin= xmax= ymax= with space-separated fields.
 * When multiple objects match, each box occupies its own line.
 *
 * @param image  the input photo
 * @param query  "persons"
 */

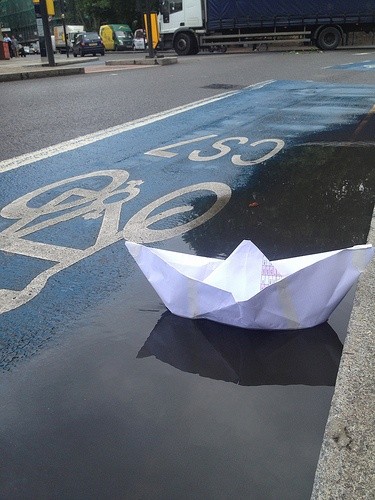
xmin=3 ymin=33 xmax=12 ymax=43
xmin=11 ymin=35 xmax=18 ymax=57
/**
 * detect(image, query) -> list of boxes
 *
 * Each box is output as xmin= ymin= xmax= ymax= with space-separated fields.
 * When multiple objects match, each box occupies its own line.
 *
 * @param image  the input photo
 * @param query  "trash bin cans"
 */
xmin=0 ymin=41 xmax=12 ymax=59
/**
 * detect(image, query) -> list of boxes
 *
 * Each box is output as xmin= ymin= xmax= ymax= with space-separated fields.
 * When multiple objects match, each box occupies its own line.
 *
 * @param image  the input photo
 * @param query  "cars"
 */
xmin=72 ymin=32 xmax=105 ymax=57
xmin=132 ymin=29 xmax=161 ymax=50
xmin=18 ymin=41 xmax=40 ymax=54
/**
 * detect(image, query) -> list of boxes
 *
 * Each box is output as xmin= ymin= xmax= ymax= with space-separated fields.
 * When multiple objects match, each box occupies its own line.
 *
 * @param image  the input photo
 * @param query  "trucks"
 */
xmin=157 ymin=0 xmax=375 ymax=56
xmin=54 ymin=25 xmax=83 ymax=54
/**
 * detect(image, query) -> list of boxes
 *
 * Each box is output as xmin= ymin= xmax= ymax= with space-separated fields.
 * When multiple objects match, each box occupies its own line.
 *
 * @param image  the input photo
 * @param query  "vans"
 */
xmin=99 ymin=25 xmax=134 ymax=50
